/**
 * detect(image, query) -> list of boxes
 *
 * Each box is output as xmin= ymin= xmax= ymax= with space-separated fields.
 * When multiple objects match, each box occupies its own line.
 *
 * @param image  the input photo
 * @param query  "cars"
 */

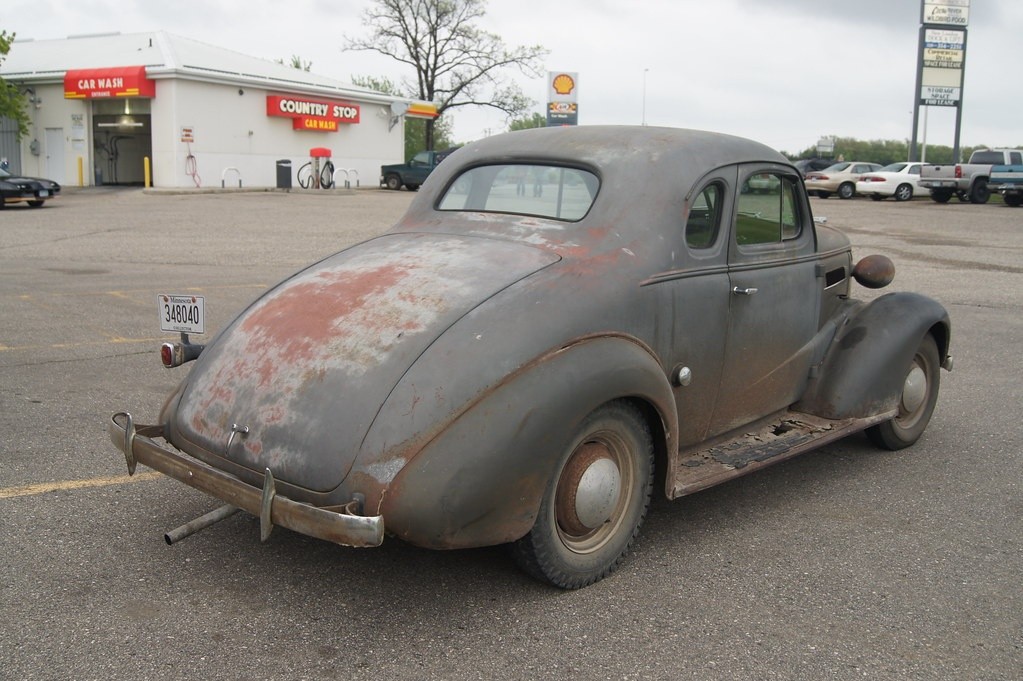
xmin=108 ymin=124 xmax=951 ymax=591
xmin=791 ymin=158 xmax=836 ymax=196
xmin=803 ymin=161 xmax=884 ymax=200
xmin=0 ymin=161 xmax=61 ymax=210
xmin=855 ymin=161 xmax=932 ymax=202
xmin=748 ymin=172 xmax=781 ymax=195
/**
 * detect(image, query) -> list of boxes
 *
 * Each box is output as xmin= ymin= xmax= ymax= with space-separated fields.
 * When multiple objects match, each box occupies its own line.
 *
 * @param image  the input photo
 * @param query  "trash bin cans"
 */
xmin=276 ymin=159 xmax=291 ymax=189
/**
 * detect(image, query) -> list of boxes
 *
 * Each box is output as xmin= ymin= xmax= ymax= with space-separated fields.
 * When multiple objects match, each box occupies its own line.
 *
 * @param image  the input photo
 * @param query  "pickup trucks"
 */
xmin=917 ymin=148 xmax=1023 ymax=204
xmin=380 ymin=147 xmax=460 ymax=191
xmin=986 ymin=164 xmax=1023 ymax=206
